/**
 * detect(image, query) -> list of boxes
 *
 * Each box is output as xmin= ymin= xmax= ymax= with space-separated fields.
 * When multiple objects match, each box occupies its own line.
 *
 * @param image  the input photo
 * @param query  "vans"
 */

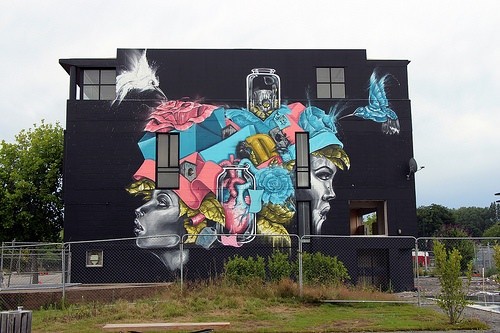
xmin=413 ymin=251 xmax=429 ymax=268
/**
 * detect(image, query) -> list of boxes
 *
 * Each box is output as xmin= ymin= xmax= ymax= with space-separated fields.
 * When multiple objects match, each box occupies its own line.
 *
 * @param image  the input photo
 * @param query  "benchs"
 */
xmin=103 ymin=322 xmax=230 ymax=333
